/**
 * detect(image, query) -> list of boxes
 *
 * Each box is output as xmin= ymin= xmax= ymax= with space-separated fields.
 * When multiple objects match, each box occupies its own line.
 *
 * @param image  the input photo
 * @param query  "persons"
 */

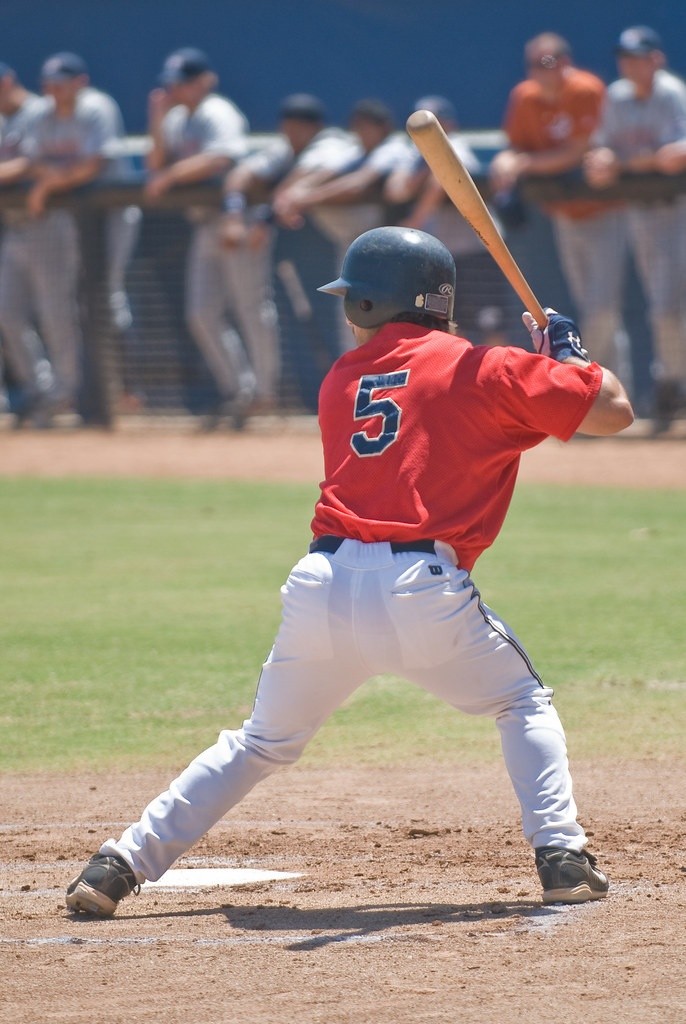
xmin=66 ymin=226 xmax=635 ymax=917
xmin=0 ymin=26 xmax=686 ymax=439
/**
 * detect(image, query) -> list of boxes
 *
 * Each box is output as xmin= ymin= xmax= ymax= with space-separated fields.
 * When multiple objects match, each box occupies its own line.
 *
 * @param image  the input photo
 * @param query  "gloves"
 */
xmin=522 ymin=307 xmax=591 ymax=363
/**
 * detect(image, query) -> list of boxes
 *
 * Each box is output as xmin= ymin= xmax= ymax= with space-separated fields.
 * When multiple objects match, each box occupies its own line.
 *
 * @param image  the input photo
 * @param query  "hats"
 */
xmin=156 ymin=48 xmax=210 ymax=85
xmin=616 ymin=25 xmax=662 ymax=55
xmin=40 ymin=52 xmax=89 ymax=79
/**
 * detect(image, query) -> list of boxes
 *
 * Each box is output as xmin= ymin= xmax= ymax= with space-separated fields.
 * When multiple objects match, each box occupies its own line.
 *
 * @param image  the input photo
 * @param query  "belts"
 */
xmin=309 ymin=535 xmax=436 ymax=554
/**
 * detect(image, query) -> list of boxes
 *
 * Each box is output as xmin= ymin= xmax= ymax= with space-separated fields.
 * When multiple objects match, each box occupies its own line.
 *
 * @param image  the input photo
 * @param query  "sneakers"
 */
xmin=535 ymin=846 xmax=609 ymax=903
xmin=65 ymin=853 xmax=141 ymax=917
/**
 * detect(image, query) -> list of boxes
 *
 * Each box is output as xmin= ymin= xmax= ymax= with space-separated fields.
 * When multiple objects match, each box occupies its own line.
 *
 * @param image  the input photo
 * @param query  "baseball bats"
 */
xmin=404 ymin=107 xmax=549 ymax=327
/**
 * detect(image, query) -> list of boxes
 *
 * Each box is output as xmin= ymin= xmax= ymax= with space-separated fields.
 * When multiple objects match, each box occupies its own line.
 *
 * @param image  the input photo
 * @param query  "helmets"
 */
xmin=316 ymin=226 xmax=457 ymax=328
